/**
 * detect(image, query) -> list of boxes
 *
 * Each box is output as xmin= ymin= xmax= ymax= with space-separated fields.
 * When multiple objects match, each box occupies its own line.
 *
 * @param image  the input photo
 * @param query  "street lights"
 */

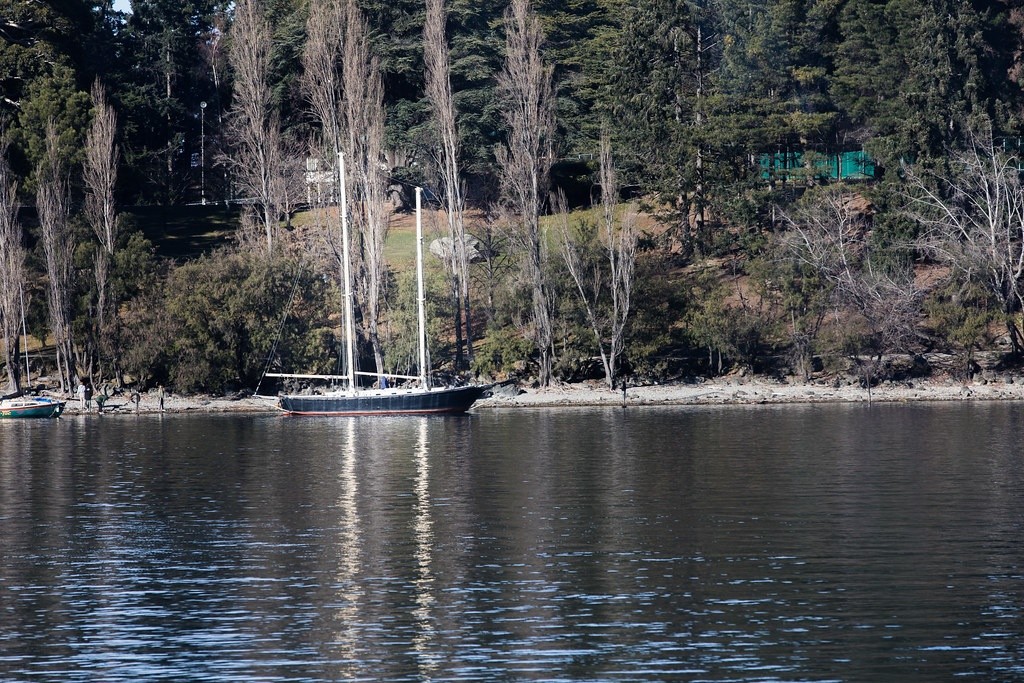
xmin=200 ymin=101 xmax=208 ymax=206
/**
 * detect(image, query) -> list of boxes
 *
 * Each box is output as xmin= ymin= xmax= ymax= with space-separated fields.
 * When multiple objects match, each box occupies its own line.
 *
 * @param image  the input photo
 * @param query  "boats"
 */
xmin=0 ymin=399 xmax=67 ymax=419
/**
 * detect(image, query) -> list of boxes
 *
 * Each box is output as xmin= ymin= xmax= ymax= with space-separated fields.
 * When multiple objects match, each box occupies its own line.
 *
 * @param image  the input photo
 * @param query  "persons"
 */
xmin=77 ymin=381 xmax=109 ymax=415
xmin=157 ymin=385 xmax=166 ymax=411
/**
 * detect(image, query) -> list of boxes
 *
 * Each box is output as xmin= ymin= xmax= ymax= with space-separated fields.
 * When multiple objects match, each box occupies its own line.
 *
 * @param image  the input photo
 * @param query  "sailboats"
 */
xmin=265 ymin=149 xmax=500 ymax=415
